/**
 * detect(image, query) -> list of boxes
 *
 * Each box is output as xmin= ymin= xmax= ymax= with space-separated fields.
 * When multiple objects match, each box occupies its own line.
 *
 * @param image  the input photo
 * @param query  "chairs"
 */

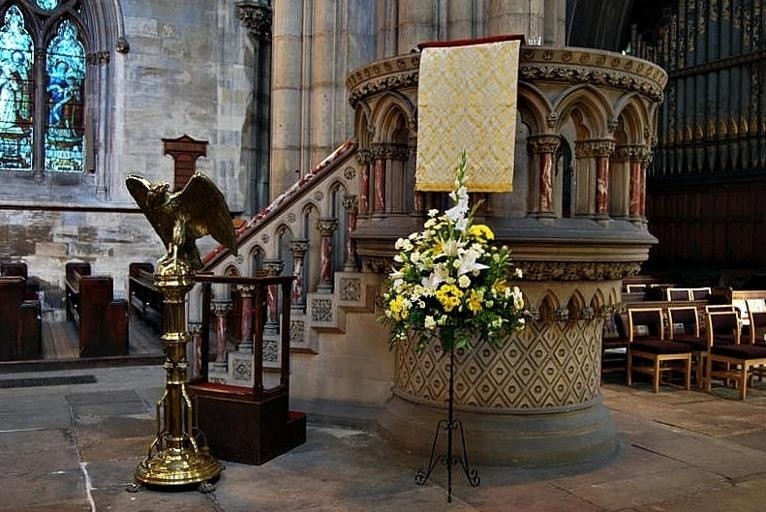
xmin=625 ymin=283 xmax=766 ymax=401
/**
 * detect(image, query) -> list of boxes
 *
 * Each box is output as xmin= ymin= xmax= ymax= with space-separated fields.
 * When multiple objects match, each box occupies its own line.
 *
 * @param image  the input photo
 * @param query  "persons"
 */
xmin=0 ymin=51 xmax=85 ymax=127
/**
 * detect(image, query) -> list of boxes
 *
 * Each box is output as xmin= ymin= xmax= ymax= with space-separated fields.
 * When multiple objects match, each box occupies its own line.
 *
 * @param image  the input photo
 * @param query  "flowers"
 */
xmin=376 ymin=153 xmax=525 ymax=360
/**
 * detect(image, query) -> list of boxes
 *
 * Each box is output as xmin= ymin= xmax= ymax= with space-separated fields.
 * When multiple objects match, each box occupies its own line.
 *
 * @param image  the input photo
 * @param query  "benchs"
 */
xmin=0 ymin=262 xmax=166 ymax=361
